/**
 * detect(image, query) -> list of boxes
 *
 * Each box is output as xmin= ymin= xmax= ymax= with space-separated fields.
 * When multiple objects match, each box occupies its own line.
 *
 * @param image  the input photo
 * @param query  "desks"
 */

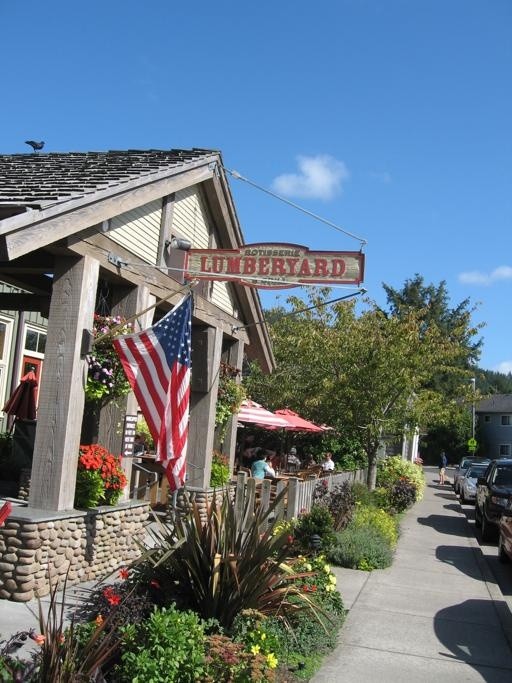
xmin=265 ymin=475 xmax=304 ymax=512
xmin=133 ymin=454 xmax=167 ymax=505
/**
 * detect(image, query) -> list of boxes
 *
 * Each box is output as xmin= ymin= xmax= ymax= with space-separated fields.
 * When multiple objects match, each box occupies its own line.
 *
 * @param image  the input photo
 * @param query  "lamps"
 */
xmin=169 ymin=236 xmax=191 ymax=251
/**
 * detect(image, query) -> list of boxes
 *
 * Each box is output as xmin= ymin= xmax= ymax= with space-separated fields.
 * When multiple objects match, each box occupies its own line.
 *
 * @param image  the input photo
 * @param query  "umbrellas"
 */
xmin=3 ymin=367 xmax=40 ymax=439
xmin=254 ymin=404 xmax=325 ymax=473
xmin=298 ymin=414 xmax=335 ymax=431
xmin=238 ymin=393 xmax=294 ymax=465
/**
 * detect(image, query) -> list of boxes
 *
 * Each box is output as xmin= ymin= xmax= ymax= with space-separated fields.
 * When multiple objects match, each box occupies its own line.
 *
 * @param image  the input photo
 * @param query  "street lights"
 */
xmin=468 ymin=377 xmax=476 ymax=457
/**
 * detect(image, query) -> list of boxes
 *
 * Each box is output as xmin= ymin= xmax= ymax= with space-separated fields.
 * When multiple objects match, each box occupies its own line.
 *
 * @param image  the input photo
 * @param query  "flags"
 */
xmin=112 ymin=286 xmax=198 ymax=492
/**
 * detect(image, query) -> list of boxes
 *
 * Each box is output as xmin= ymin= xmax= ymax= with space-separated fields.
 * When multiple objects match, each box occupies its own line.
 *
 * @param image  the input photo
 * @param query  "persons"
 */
xmin=250 ymin=445 xmax=335 ymax=480
xmin=437 ymin=451 xmax=448 ymax=485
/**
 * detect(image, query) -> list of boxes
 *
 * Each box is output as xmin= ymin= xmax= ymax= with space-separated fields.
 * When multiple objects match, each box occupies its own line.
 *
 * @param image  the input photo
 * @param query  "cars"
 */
xmin=451 ymin=454 xmax=512 ymax=558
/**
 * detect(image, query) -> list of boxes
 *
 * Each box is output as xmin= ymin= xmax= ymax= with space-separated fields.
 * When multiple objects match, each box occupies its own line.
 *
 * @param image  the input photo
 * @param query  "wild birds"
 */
xmin=25 ymin=140 xmax=45 ymax=153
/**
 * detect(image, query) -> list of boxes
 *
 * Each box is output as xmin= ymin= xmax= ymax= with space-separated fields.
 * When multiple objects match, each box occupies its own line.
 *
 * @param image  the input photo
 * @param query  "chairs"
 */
xmin=239 ymin=466 xmax=272 ymax=500
xmin=297 ymin=465 xmax=323 ymax=482
